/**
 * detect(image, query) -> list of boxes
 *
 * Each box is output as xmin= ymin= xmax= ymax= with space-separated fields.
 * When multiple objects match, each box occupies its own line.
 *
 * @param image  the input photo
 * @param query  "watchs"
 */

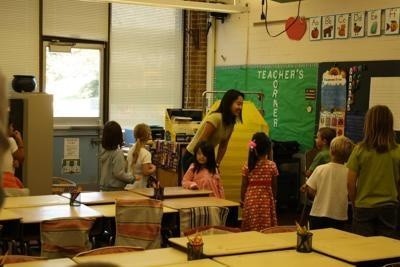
xmin=216 ymin=163 xmax=220 ymax=167
xmin=17 ymin=145 xmax=25 ymax=148
xmin=240 ymin=200 xmax=245 ymax=204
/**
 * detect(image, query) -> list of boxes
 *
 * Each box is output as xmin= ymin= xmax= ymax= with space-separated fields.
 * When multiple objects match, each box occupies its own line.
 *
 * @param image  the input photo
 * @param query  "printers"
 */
xmin=164 ymin=108 xmax=205 ymax=145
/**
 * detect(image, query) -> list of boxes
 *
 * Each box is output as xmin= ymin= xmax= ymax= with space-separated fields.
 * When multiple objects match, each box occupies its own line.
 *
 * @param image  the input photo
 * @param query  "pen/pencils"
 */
xmin=69 ymin=186 xmax=81 ymax=206
xmin=296 ymin=219 xmax=311 ymax=252
xmin=187 ymin=230 xmax=204 ymax=258
xmin=153 ymin=181 xmax=163 ymax=201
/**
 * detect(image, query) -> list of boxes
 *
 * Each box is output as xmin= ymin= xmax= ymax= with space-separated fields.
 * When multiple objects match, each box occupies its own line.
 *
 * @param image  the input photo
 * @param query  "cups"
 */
xmin=187 ymin=243 xmax=204 ymax=260
xmin=296 ymin=233 xmax=312 ymax=252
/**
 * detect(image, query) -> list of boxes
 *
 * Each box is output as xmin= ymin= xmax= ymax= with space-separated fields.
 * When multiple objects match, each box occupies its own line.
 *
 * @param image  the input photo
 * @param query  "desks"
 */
xmin=72 ymin=245 xmax=193 ymax=265
xmin=168 ymin=229 xmax=288 ymax=257
xmin=0 ymin=207 xmax=23 ymax=222
xmin=211 ymin=249 xmax=355 ymax=267
xmin=166 ymin=256 xmax=228 ymax=266
xmin=4 ymin=256 xmax=77 ymax=267
xmin=263 ymin=226 xmax=363 ymax=248
xmin=88 ymin=201 xmax=180 ymax=220
xmin=163 ymin=195 xmax=244 ymax=215
xmin=62 ymin=190 xmax=145 ymax=208
xmin=315 ymin=234 xmax=400 ymax=264
xmin=134 ymin=185 xmax=211 ymax=200
xmin=13 ymin=203 xmax=102 ymax=228
xmin=2 ymin=192 xmax=79 ymax=209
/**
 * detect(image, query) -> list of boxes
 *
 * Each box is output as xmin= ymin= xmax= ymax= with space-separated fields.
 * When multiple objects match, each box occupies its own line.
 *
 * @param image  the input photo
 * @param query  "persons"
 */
xmin=96 ymin=121 xmax=142 ymax=192
xmin=124 ymin=123 xmax=156 ymax=191
xmin=0 ymin=121 xmax=25 ymax=189
xmin=177 ymin=89 xmax=245 ymax=186
xmin=346 ymin=105 xmax=400 ymax=267
xmin=306 ymin=135 xmax=359 ymax=230
xmin=239 ymin=132 xmax=280 ymax=231
xmin=304 ymin=128 xmax=336 ymax=178
xmin=181 ymin=141 xmax=225 ymax=200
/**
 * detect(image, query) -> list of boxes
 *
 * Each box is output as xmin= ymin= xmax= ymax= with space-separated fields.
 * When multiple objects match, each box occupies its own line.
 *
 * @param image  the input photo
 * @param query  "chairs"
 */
xmin=185 ymin=225 xmax=240 ymax=235
xmin=261 ymin=224 xmax=306 ymax=233
xmin=73 ymin=245 xmax=143 ymax=256
xmin=177 ymin=206 xmax=232 ymax=233
xmin=0 ymin=255 xmax=46 ymax=265
xmin=41 ymin=217 xmax=93 ymax=253
xmin=114 ymin=197 xmax=163 ymax=247
